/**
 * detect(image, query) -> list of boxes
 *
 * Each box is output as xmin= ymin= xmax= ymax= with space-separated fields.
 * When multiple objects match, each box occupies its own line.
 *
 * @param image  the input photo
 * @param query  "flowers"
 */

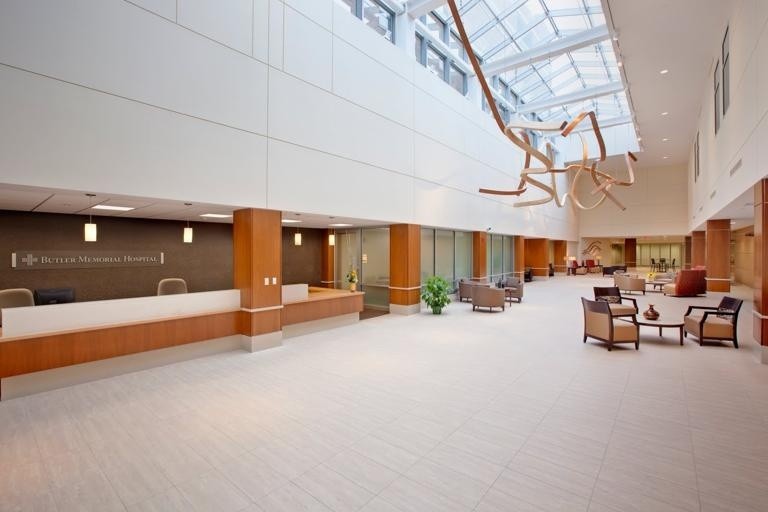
xmin=346 ymin=260 xmax=360 ymax=283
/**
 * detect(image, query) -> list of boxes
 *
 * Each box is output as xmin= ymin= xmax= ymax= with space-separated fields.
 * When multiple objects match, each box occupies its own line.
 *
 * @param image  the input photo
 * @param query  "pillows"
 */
xmin=597 ymin=294 xmax=620 ymax=304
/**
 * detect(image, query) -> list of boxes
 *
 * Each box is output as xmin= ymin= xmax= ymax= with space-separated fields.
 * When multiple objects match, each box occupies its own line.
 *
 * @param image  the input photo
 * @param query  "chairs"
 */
xmin=649 ymin=256 xmax=676 ymax=274
xmin=592 ymin=286 xmax=640 ymax=316
xmin=0 ymin=288 xmax=40 ymax=308
xmin=564 ymin=256 xmax=627 ymax=277
xmin=579 ymin=295 xmax=641 ymax=351
xmin=681 ymin=295 xmax=743 ymax=349
xmin=157 ymin=277 xmax=189 ymax=296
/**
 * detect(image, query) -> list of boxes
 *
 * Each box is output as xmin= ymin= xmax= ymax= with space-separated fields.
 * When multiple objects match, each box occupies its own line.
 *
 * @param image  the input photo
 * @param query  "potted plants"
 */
xmin=419 ymin=275 xmax=454 ymax=315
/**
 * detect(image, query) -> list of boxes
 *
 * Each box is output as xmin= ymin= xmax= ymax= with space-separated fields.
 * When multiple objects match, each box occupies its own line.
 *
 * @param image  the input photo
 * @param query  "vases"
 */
xmin=350 ymin=281 xmax=356 ymax=293
xmin=643 ymin=302 xmax=660 ymax=320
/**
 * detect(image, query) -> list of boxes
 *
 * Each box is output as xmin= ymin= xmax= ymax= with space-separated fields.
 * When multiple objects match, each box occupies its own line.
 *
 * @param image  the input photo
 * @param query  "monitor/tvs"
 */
xmin=34 ymin=288 xmax=74 ymax=305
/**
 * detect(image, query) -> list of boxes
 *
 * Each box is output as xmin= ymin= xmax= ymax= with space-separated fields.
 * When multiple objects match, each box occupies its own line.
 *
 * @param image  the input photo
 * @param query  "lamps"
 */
xmin=82 ymin=193 xmax=99 ymax=244
xmin=293 ymin=214 xmax=302 ymax=246
xmin=183 ymin=203 xmax=195 ymax=244
xmin=327 ymin=215 xmax=336 ymax=245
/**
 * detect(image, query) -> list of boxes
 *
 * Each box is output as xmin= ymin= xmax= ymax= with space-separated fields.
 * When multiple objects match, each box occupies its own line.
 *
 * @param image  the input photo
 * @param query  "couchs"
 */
xmin=610 ymin=269 xmax=710 ymax=298
xmin=458 ymin=273 xmax=525 ymax=314
xmin=524 ymin=263 xmax=554 ymax=282
